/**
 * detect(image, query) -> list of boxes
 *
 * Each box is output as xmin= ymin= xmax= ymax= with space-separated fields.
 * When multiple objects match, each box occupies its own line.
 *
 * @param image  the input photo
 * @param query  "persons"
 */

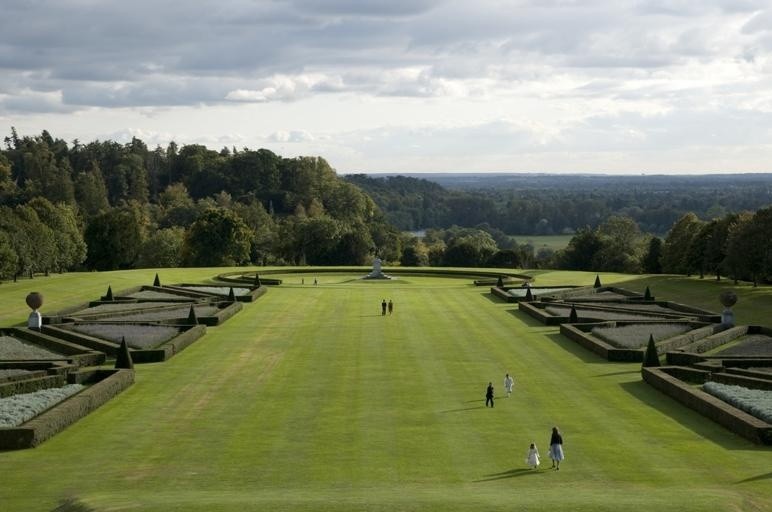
xmin=381 ymin=299 xmax=387 ymax=317
xmin=503 ymin=373 xmax=515 ymax=398
xmin=388 ymin=299 xmax=393 ymax=317
xmin=547 ymin=427 xmax=564 ymax=471
xmin=485 ymin=382 xmax=494 ymax=408
xmin=525 ymin=443 xmax=540 ymax=470
